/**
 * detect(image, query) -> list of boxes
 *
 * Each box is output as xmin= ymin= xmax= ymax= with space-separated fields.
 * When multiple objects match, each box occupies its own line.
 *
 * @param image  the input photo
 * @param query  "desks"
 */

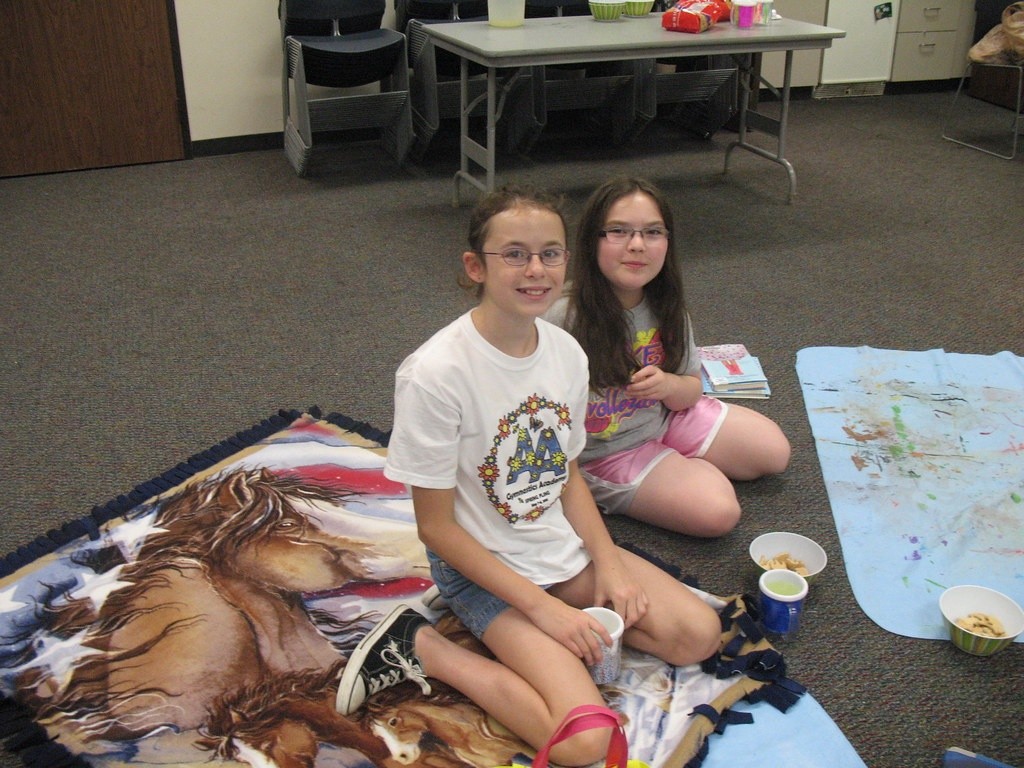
xmin=420 ymin=8 xmax=848 ymax=216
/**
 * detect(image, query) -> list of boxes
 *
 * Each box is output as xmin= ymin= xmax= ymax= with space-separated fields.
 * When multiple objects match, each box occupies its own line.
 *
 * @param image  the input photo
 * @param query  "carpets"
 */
xmin=0 ymin=403 xmax=783 ymax=768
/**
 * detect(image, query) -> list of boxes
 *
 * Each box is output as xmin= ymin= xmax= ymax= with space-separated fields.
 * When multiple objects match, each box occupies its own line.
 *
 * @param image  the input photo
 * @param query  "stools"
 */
xmin=939 ymin=63 xmax=1023 ymax=160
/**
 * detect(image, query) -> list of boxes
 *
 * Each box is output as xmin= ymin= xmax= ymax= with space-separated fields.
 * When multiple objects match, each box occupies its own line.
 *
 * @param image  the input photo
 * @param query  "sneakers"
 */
xmin=335 ymin=602 xmax=433 ymax=720
xmin=421 ymin=583 xmax=450 ymax=610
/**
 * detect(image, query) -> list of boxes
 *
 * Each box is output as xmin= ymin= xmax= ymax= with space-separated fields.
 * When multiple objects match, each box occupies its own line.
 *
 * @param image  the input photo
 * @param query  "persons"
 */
xmin=335 ymin=185 xmax=722 ymax=768
xmin=536 ymin=173 xmax=791 ymax=540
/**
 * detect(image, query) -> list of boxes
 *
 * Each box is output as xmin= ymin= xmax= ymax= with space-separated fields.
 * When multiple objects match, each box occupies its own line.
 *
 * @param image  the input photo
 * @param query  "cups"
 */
xmin=730 ymin=0 xmax=773 ymax=27
xmin=759 ymin=569 xmax=809 ymax=636
xmin=579 ymin=608 xmax=624 ymax=685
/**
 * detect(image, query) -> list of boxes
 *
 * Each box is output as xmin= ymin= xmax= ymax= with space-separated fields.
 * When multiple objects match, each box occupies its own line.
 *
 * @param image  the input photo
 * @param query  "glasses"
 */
xmin=477 ymin=248 xmax=572 ymax=267
xmin=598 ymin=226 xmax=669 ymax=244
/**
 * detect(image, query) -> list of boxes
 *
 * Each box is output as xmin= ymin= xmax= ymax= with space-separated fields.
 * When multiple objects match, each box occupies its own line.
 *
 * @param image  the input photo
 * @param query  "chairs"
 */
xmin=276 ymin=0 xmax=740 ymax=176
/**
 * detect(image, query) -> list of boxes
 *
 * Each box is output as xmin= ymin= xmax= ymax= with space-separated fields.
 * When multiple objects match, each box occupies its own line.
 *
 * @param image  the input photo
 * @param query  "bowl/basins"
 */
xmin=940 ymin=584 xmax=1024 ymax=658
xmin=588 ymin=0 xmax=625 ymax=22
xmin=749 ymin=532 xmax=827 ymax=588
xmin=623 ymin=0 xmax=654 ymax=17
xmin=487 ymin=0 xmax=527 ymax=26
